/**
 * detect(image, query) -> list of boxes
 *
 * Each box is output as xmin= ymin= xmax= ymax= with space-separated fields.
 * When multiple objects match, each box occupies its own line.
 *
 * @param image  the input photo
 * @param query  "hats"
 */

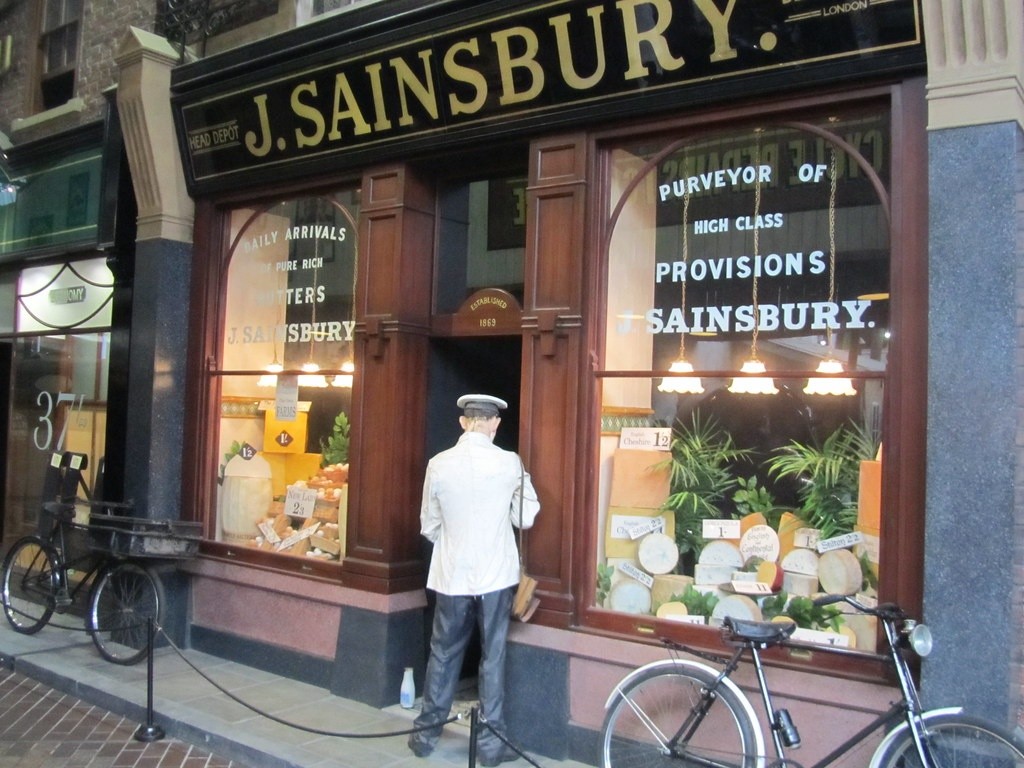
xmin=457 ymin=394 xmax=507 ymax=410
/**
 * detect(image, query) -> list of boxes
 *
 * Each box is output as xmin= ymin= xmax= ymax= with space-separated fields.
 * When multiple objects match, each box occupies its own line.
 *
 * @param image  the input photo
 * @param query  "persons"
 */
xmin=408 ymin=392 xmax=540 ymax=767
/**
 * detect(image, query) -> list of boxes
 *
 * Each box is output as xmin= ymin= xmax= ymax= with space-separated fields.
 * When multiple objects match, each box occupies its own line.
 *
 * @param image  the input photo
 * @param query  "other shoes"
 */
xmin=408 ymin=737 xmax=429 ymax=757
xmin=483 ymin=744 xmax=522 ymax=766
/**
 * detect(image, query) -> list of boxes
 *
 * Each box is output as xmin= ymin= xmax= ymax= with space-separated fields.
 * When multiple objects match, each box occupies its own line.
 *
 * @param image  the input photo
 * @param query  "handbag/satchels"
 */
xmin=512 ymin=574 xmax=541 ymax=623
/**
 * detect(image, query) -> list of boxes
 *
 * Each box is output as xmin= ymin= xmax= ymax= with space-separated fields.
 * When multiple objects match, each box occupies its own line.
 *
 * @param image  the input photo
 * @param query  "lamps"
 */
xmin=257 ymin=201 xmax=290 ymax=386
xmin=331 ymin=204 xmax=361 ymax=388
xmin=657 ymin=145 xmax=706 ymax=395
xmin=803 ymin=116 xmax=858 ymax=397
xmin=300 ymin=197 xmax=330 ymax=388
xmin=728 ymin=128 xmax=780 ymax=395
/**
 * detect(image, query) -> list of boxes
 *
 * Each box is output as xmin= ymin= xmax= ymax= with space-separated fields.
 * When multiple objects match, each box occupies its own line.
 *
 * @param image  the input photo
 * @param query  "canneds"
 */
xmin=774 ymin=709 xmax=800 ymax=746
